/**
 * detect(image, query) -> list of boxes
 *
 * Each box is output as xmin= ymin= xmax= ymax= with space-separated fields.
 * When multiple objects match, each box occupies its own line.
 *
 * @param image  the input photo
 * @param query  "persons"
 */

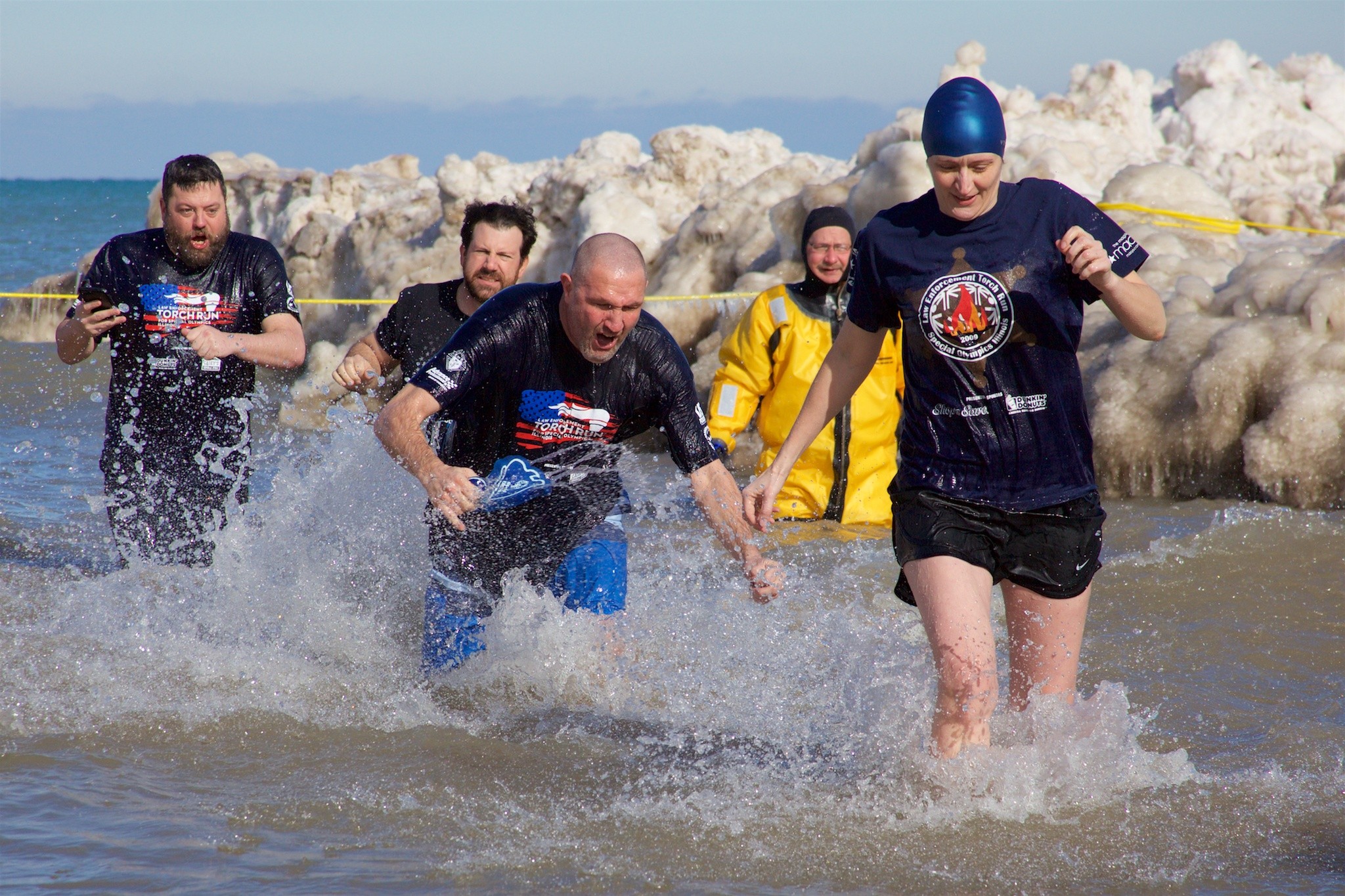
xmin=738 ymin=76 xmax=1166 ymax=759
xmin=372 ymin=233 xmax=784 ymax=672
xmin=55 ymin=154 xmax=310 ymax=567
xmin=329 ymin=203 xmax=537 ymax=389
xmin=706 ymin=204 xmax=908 ymax=524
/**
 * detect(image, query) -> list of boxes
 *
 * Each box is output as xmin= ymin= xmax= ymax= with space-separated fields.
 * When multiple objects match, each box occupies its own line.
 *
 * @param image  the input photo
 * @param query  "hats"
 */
xmin=922 ymin=76 xmax=1006 ymax=158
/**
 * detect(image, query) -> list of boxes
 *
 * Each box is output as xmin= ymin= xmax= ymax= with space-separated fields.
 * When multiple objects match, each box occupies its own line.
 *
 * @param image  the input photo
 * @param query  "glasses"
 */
xmin=808 ymin=241 xmax=853 ymax=254
